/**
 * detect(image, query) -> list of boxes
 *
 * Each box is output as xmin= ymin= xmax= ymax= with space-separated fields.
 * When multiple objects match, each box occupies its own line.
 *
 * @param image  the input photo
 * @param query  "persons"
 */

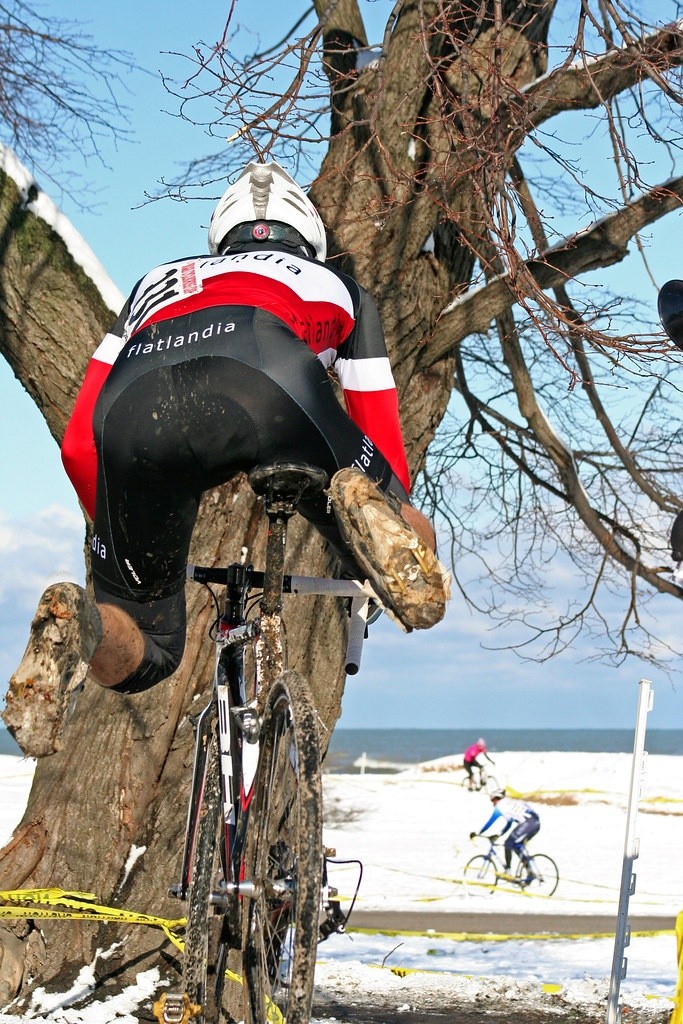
xmin=3 ymin=161 xmax=444 ymax=758
xmin=470 ymin=789 xmax=540 ymax=886
xmin=464 ymin=738 xmax=495 ymax=792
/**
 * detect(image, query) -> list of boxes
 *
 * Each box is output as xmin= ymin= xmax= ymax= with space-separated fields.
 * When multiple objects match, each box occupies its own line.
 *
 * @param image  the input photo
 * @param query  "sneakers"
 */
xmin=329 ymin=466 xmax=450 ymax=631
xmin=4 ymin=582 xmax=103 ymax=758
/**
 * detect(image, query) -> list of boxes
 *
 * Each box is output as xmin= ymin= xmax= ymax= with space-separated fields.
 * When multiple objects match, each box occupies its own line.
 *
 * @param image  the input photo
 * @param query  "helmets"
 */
xmin=489 ymin=787 xmax=506 ymax=800
xmin=206 ymin=161 xmax=326 ymax=265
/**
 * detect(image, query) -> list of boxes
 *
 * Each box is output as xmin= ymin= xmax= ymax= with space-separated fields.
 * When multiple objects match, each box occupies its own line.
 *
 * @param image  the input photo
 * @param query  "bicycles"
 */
xmin=183 ymin=463 xmax=378 ymax=1023
xmin=464 ymin=833 xmax=560 ymax=897
xmin=461 ymin=763 xmax=500 ymax=795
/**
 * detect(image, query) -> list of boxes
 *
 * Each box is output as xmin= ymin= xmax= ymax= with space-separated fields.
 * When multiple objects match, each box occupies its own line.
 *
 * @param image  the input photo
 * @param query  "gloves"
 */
xmin=469 ymin=832 xmax=476 ymax=839
xmin=490 ymin=834 xmax=498 ymax=841
